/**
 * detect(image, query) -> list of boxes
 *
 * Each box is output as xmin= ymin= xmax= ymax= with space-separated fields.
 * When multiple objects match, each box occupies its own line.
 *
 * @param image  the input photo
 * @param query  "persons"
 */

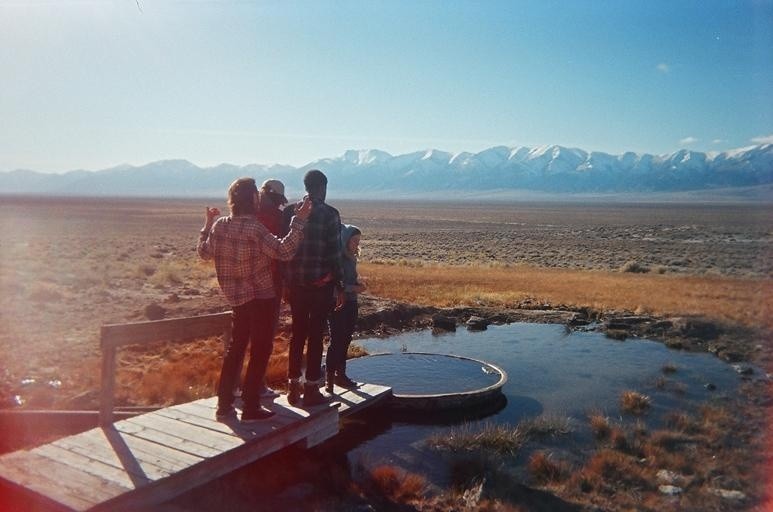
xmin=281 ymin=168 xmax=345 ymax=407
xmin=231 ymin=178 xmax=288 ymax=398
xmin=321 ymin=222 xmax=367 ymax=392
xmin=197 ymin=175 xmax=313 ymax=424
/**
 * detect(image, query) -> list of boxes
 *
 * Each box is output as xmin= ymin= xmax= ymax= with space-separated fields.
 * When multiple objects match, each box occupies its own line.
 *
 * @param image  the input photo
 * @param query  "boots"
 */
xmin=287 ymin=366 xmax=358 ymax=408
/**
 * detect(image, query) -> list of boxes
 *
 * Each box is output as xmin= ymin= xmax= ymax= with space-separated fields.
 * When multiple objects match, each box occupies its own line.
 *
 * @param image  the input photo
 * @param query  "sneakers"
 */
xmin=214 ymin=386 xmax=281 ymax=424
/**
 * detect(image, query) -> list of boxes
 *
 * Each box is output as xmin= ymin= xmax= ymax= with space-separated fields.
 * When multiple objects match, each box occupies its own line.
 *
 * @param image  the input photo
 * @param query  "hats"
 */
xmin=261 ymin=178 xmax=289 ymax=204
